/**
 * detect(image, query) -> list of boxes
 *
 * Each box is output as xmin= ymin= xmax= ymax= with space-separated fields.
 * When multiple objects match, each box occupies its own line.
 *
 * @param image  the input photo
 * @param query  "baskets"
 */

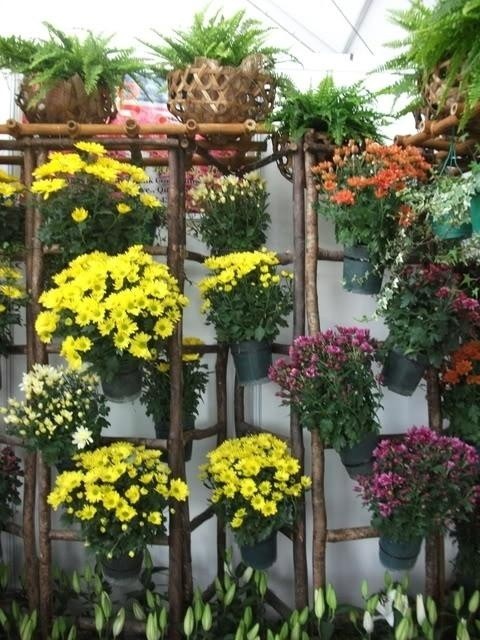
xmin=14 ymin=70 xmax=118 ymax=124
xmin=416 ymin=56 xmax=473 ymax=118
xmin=272 ymin=131 xmax=347 ymax=184
xmin=166 ymin=63 xmax=277 ymax=123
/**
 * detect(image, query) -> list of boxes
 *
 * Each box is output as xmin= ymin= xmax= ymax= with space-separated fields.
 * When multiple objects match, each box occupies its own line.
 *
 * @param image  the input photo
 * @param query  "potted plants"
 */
xmin=0 ymin=0 xmax=480 ymax=187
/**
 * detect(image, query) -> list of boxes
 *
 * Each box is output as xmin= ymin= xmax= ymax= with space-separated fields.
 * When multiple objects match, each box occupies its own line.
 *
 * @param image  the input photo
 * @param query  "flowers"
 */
xmin=0 ymin=137 xmax=480 ymax=640
